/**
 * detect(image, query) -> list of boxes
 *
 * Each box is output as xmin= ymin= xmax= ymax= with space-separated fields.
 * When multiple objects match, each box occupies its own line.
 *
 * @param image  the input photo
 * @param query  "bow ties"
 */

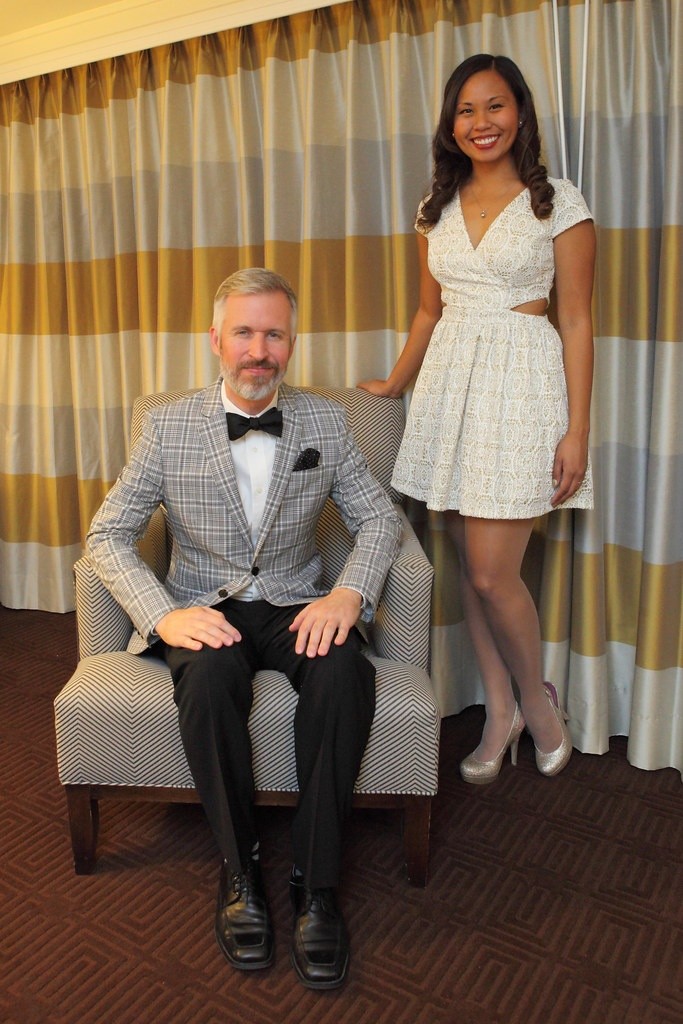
xmin=226 ymin=406 xmax=283 ymax=441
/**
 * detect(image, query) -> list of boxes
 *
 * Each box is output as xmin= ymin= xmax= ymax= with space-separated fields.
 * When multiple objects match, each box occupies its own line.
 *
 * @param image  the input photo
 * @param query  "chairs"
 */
xmin=53 ymin=387 xmax=441 ymax=889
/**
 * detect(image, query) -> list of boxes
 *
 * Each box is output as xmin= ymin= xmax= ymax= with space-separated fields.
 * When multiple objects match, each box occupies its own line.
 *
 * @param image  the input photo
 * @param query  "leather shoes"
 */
xmin=288 ymin=864 xmax=351 ymax=990
xmin=215 ymin=850 xmax=275 ymax=970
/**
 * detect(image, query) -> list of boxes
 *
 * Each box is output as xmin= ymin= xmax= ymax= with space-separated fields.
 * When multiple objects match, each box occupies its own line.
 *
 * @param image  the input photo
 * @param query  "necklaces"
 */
xmin=470 ymin=185 xmax=514 ymax=218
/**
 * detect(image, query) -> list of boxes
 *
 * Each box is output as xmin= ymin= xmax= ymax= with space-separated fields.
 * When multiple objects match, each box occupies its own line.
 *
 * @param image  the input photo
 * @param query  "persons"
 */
xmin=355 ymin=53 xmax=596 ymax=788
xmin=84 ymin=267 xmax=405 ymax=990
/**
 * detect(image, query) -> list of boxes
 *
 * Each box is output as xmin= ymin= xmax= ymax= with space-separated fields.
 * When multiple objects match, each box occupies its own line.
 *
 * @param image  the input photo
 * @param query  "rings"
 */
xmin=578 ymin=481 xmax=582 ymax=483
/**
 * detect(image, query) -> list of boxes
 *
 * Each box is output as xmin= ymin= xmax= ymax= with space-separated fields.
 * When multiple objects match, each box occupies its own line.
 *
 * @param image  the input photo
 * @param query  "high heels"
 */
xmin=460 ymin=701 xmax=525 ymax=785
xmin=533 ymin=682 xmax=573 ymax=776
